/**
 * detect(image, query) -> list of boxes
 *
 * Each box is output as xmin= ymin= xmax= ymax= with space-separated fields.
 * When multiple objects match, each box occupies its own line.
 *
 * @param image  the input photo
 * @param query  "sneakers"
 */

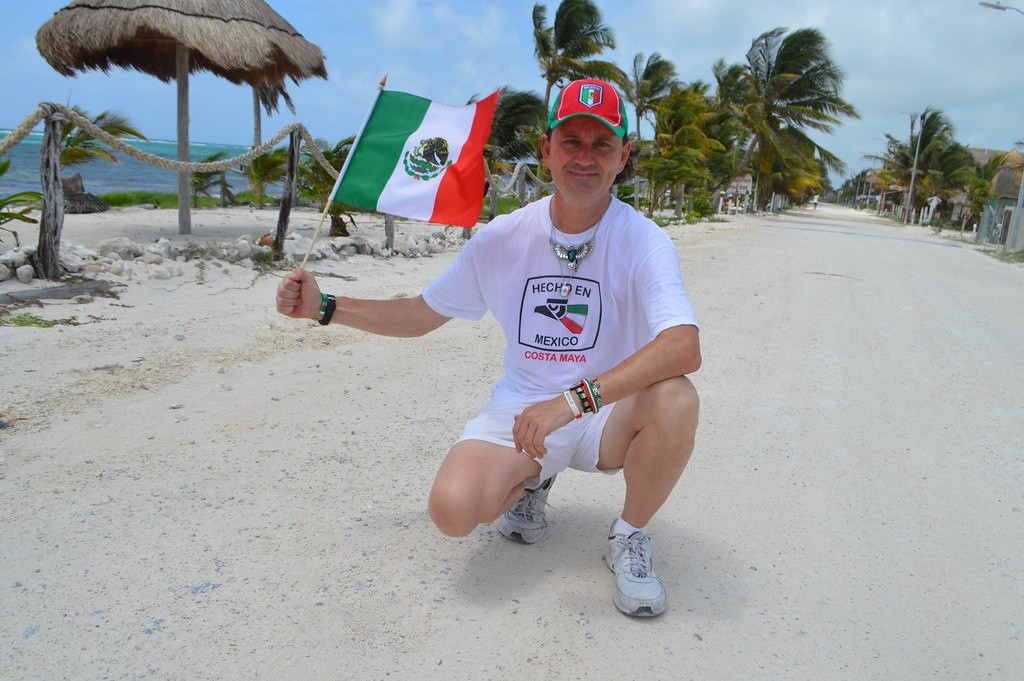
xmin=498 ymin=473 xmax=557 ymax=544
xmin=606 ymin=518 xmax=667 ymax=618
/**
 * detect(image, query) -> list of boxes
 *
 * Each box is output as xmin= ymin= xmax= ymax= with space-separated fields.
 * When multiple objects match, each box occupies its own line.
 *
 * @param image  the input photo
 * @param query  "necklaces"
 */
xmin=549 ymin=197 xmax=611 ymax=297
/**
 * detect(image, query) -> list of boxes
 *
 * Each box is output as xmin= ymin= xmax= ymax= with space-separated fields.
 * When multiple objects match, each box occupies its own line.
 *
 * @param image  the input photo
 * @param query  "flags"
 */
xmin=334 ymin=90 xmax=498 ymax=227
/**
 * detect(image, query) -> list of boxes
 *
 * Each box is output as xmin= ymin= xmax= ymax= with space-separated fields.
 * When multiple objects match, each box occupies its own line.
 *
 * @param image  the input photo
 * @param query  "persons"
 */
xmin=277 ymin=79 xmax=702 ymax=617
xmin=814 ymin=194 xmax=819 ymax=209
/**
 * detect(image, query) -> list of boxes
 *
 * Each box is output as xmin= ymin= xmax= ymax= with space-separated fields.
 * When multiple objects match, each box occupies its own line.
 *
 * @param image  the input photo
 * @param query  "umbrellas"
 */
xmin=35 ymin=0 xmax=327 ymax=235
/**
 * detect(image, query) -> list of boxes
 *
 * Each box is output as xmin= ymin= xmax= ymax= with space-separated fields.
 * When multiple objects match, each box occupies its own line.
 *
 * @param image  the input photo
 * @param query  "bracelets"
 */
xmin=313 ymin=293 xmax=336 ymax=326
xmin=564 ymin=377 xmax=603 ymax=418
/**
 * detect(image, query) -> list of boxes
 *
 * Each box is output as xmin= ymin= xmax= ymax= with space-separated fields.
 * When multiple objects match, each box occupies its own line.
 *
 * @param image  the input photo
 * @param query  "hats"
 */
xmin=547 ymin=78 xmax=628 ymax=137
